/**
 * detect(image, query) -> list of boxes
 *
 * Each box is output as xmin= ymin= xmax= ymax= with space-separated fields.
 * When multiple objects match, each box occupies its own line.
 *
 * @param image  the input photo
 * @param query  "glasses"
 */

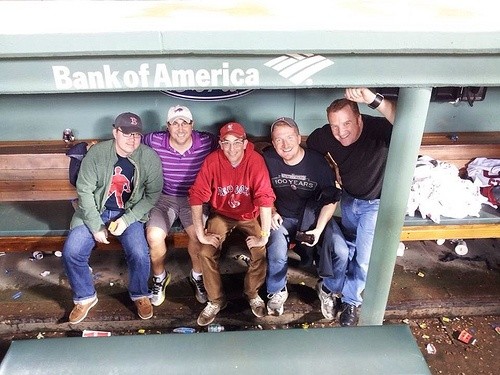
xmin=116 ymin=128 xmax=142 ymax=138
xmin=220 ymin=138 xmax=243 ymax=146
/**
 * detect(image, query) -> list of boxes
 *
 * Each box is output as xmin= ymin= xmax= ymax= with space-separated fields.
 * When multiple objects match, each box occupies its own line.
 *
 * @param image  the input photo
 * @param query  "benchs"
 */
xmin=0 ymin=138 xmax=500 ymax=253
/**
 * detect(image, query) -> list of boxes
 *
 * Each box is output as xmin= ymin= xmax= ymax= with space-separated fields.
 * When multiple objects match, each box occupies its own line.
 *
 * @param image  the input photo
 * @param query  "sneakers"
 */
xmin=267 ymin=285 xmax=289 ymax=316
xmin=315 ymin=279 xmax=336 ymax=320
xmin=150 ymin=271 xmax=172 ymax=307
xmin=248 ymin=294 xmax=267 ymax=318
xmin=68 ymin=299 xmax=99 ymax=324
xmin=188 ymin=269 xmax=207 ymax=304
xmin=134 ymin=297 xmax=154 ymax=320
xmin=197 ymin=298 xmax=228 ymax=326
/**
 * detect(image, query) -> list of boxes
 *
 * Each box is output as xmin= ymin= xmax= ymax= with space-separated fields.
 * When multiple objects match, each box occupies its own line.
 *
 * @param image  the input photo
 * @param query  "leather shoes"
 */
xmin=340 ymin=301 xmax=357 ymax=327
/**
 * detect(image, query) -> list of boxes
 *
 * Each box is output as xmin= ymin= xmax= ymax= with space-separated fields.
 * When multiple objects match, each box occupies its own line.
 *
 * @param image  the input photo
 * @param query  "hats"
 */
xmin=271 ymin=118 xmax=298 ymax=134
xmin=220 ymin=123 xmax=247 ymax=140
xmin=113 ymin=112 xmax=144 ymax=137
xmin=166 ymin=106 xmax=193 ymax=124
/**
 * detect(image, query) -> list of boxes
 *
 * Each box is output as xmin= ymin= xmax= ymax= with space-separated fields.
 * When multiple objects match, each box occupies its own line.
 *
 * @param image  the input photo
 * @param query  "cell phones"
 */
xmin=108 ymin=221 xmax=118 ymax=231
xmin=295 ymin=231 xmax=315 ymax=245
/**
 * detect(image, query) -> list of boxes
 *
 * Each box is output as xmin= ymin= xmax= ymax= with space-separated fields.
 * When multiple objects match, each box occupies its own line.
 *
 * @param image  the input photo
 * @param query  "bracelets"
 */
xmin=368 ymin=93 xmax=384 ymax=110
xmin=260 ymin=231 xmax=270 ymax=237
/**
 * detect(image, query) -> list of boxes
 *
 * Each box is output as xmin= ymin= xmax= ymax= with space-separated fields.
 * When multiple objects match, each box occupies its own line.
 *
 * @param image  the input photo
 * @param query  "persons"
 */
xmin=188 ymin=122 xmax=276 ymax=326
xmin=258 ymin=117 xmax=350 ymax=321
xmin=141 ymin=105 xmax=220 ymax=305
xmin=306 ymin=88 xmax=397 ymax=326
xmin=62 ymin=113 xmax=164 ymax=324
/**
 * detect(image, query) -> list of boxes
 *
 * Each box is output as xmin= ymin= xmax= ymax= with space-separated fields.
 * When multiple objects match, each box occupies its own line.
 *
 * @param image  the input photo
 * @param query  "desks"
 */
xmin=0 ymin=324 xmax=431 ymax=375
